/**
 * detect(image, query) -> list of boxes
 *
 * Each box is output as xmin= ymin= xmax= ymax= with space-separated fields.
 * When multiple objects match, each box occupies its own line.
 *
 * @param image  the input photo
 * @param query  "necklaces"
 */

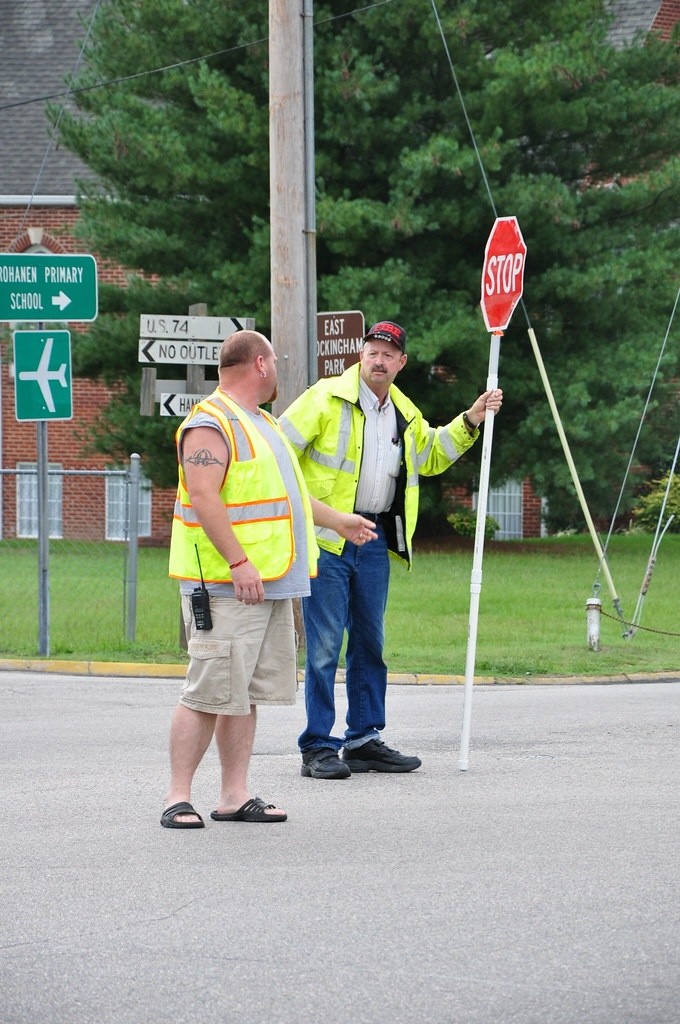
xmin=219 ymin=390 xmax=237 ymax=403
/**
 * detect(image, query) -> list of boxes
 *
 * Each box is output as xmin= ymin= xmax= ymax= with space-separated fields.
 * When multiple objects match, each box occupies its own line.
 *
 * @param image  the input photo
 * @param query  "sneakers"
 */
xmin=342 ymin=736 xmax=422 ymax=773
xmin=301 ymin=747 xmax=352 ymax=778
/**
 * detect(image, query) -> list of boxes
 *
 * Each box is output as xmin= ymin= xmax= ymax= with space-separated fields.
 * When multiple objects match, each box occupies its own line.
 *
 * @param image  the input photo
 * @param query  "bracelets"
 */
xmin=230 ymin=557 xmax=248 ymax=568
xmin=463 ymin=413 xmax=480 ymax=430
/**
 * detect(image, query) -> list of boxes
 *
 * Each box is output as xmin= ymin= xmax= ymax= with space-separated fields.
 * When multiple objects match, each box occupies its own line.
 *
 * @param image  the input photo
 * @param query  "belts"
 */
xmin=355 ymin=513 xmax=384 ymax=525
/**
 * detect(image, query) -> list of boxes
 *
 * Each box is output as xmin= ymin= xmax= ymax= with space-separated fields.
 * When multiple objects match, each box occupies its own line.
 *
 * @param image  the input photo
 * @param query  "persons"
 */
xmin=160 ymin=330 xmax=378 ymax=829
xmin=280 ymin=321 xmax=504 ymax=779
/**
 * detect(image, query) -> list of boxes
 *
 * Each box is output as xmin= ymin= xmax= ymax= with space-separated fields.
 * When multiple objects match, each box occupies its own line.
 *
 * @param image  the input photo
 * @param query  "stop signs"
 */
xmin=480 ymin=216 xmax=527 ymax=332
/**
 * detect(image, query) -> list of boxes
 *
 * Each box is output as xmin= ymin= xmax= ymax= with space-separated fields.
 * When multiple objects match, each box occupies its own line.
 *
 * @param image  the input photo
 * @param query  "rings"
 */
xmin=359 ymin=533 xmax=362 ymax=538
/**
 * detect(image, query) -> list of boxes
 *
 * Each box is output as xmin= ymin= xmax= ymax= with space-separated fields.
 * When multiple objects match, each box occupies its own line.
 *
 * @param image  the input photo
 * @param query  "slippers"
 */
xmin=160 ymin=800 xmax=204 ymax=828
xmin=211 ymin=795 xmax=287 ymax=822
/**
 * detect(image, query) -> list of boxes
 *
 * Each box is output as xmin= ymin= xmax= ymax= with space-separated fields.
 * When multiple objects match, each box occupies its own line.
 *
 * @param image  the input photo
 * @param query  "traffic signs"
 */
xmin=138 ymin=340 xmax=223 ymax=366
xmin=0 ymin=253 xmax=98 ymax=323
xmin=139 ymin=313 xmax=256 ymax=341
xmin=160 ymin=393 xmax=209 ymax=417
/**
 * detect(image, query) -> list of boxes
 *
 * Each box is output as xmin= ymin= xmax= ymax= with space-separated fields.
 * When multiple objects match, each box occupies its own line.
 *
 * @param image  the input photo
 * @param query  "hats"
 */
xmin=364 ymin=320 xmax=406 ymax=355
